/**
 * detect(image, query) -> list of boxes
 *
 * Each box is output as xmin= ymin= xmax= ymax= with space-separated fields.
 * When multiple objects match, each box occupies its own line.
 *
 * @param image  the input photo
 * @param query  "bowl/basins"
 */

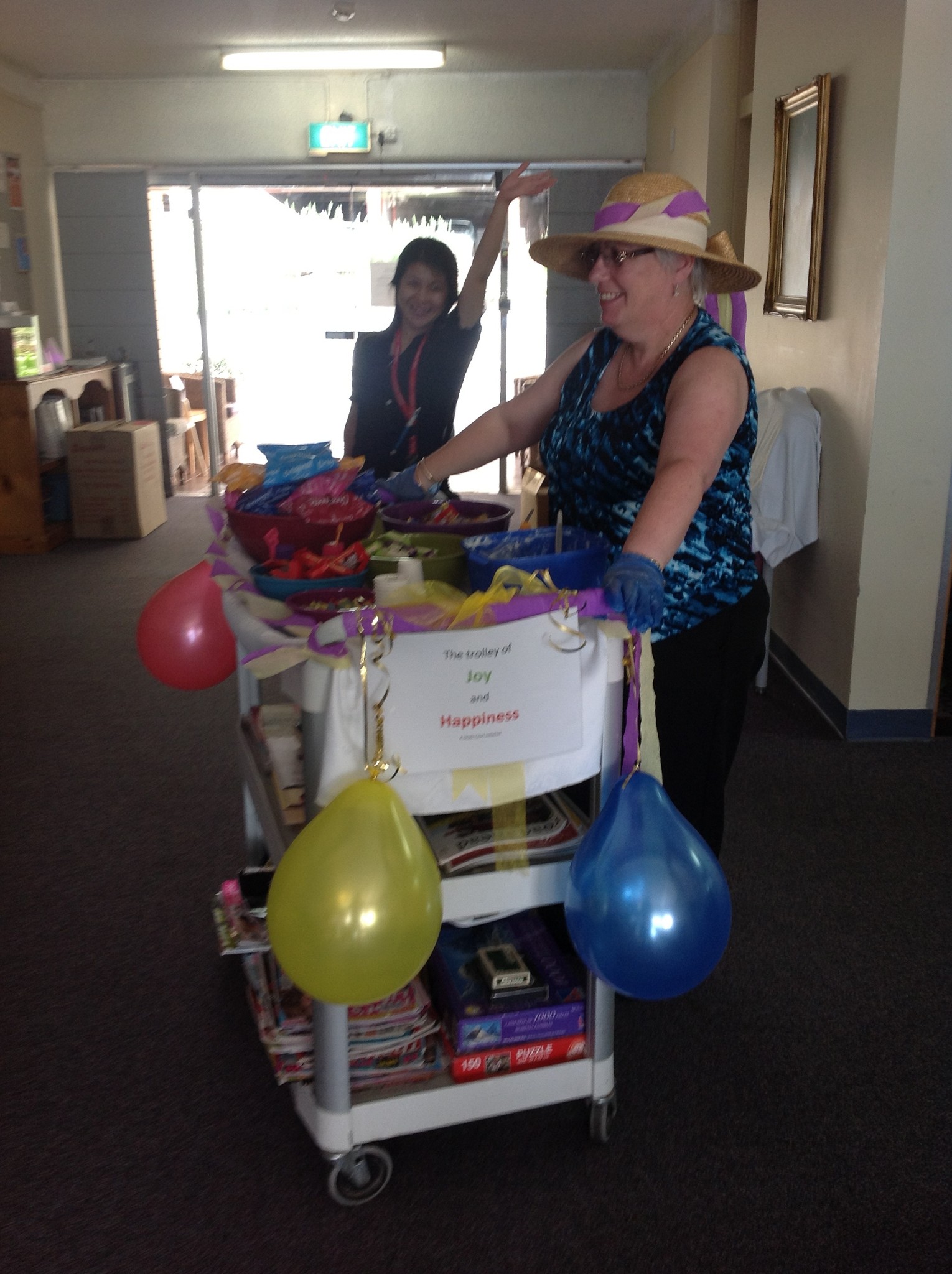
xmin=222 ymin=468 xmax=604 ymax=627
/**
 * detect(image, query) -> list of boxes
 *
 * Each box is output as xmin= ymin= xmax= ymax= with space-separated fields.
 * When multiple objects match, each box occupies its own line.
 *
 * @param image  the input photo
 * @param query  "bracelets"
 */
xmin=416 ymin=457 xmax=441 ymax=495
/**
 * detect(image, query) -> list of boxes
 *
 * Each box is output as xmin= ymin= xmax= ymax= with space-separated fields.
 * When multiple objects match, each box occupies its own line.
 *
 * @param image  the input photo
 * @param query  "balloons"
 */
xmin=135 ymin=556 xmax=237 ymax=691
xmin=564 ymin=771 xmax=735 ymax=1001
xmin=265 ymin=774 xmax=446 ymax=1006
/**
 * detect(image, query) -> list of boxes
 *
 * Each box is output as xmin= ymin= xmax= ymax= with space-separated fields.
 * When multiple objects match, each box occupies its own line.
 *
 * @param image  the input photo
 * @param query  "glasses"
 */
xmin=581 ymin=244 xmax=657 ymax=270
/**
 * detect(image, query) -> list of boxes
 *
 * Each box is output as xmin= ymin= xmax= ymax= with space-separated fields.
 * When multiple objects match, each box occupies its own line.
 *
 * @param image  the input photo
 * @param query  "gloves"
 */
xmin=375 ymin=464 xmax=429 ymax=500
xmin=605 ymin=552 xmax=667 ymax=634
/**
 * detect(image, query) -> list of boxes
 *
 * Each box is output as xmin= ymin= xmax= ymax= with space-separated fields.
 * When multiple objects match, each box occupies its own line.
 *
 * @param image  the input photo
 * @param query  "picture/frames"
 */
xmin=759 ymin=70 xmax=830 ymax=324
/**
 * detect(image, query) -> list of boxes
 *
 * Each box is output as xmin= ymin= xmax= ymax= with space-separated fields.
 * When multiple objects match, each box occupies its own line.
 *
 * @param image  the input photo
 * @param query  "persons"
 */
xmin=389 ymin=170 xmax=774 ymax=875
xmin=341 ymin=157 xmax=559 ymax=500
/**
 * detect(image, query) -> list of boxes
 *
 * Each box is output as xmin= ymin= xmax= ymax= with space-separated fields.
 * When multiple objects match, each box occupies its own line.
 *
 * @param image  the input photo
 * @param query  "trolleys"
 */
xmin=206 ymin=496 xmax=636 ymax=1207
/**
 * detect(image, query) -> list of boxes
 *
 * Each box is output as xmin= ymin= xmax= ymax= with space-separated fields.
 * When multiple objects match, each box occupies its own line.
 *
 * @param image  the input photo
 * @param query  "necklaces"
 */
xmin=615 ymin=303 xmax=700 ymax=389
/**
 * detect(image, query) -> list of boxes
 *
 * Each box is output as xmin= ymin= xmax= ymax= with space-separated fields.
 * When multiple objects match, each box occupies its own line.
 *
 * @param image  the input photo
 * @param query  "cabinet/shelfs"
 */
xmin=0 ymin=360 xmax=123 ymax=555
xmin=204 ymin=529 xmax=636 ymax=1209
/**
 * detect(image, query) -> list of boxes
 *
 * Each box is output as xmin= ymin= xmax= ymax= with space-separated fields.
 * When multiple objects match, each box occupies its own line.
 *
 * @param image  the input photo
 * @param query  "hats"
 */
xmin=528 ymin=170 xmax=761 ymax=293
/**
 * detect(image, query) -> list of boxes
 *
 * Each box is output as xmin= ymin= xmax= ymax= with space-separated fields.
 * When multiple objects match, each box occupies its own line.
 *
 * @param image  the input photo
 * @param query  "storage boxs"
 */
xmin=69 ymin=418 xmax=170 ymax=540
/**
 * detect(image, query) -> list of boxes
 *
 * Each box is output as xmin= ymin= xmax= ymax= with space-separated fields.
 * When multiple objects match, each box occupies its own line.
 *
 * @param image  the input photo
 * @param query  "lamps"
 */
xmin=216 ymin=43 xmax=447 ymax=74
